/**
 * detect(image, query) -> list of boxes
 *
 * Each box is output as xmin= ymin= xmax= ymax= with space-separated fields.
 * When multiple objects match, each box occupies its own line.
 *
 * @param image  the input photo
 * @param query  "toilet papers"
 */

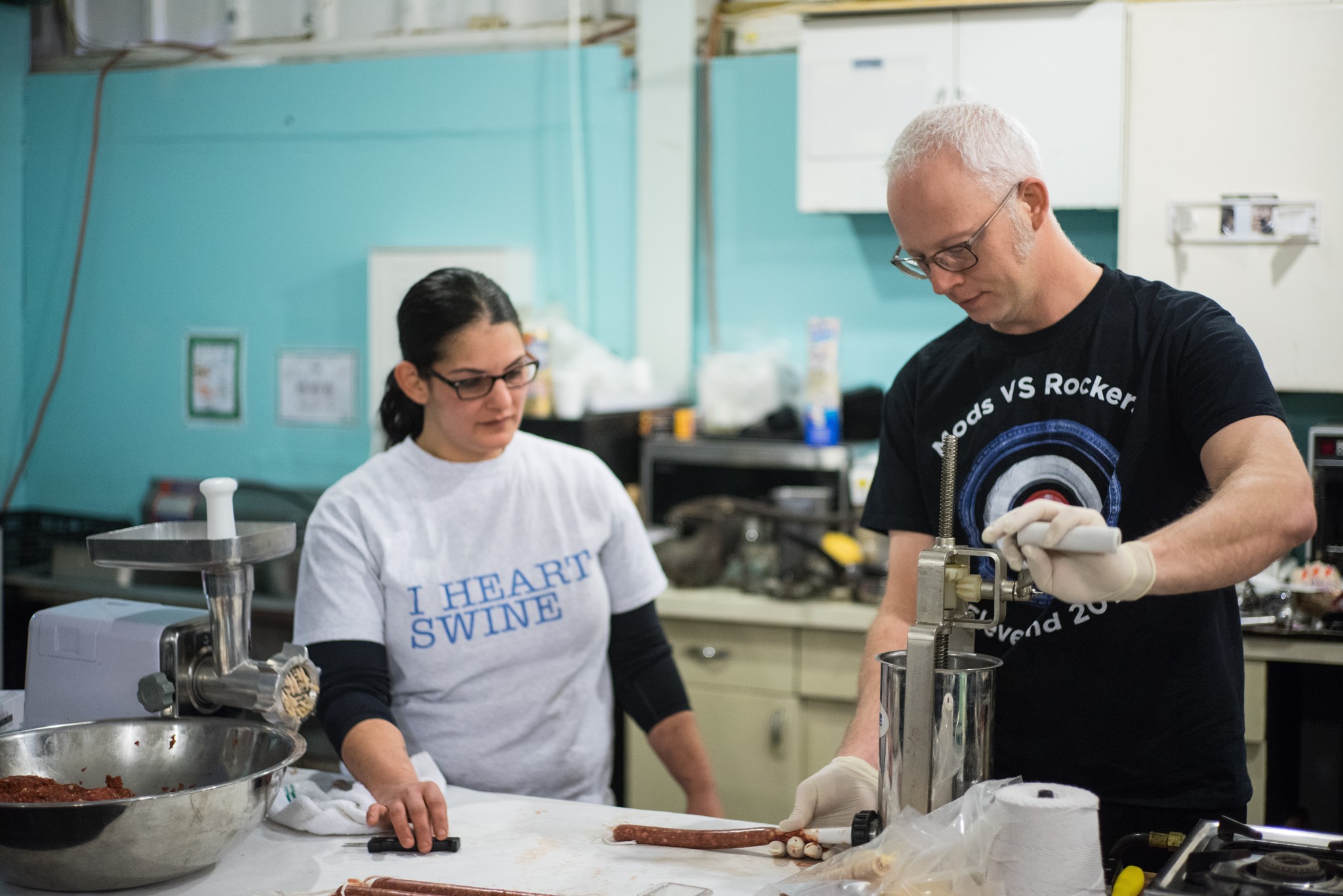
xmin=986 ymin=780 xmax=1107 ymax=896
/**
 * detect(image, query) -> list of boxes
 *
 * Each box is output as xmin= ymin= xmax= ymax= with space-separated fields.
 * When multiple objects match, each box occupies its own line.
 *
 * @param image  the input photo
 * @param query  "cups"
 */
xmin=673 ymin=407 xmax=696 ymax=440
xmin=878 ymin=648 xmax=1001 ymax=836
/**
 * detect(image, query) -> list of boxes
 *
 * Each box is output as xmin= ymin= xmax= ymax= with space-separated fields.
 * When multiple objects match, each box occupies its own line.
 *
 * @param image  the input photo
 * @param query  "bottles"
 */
xmin=808 ymin=315 xmax=843 ymax=447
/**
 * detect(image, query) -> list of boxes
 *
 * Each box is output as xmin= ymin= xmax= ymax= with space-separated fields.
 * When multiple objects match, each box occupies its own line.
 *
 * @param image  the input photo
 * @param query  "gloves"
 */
xmin=980 ymin=499 xmax=1158 ymax=604
xmin=768 ymin=757 xmax=885 ymax=861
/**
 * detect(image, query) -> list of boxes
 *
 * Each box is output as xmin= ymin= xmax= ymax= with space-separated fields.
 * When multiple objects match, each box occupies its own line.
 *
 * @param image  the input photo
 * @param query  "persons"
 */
xmin=771 ymin=103 xmax=1317 ymax=860
xmin=292 ymin=268 xmax=720 ymax=853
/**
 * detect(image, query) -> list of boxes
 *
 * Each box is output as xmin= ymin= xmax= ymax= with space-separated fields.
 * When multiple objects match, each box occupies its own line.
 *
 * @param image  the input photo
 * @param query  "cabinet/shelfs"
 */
xmin=800 ymin=0 xmax=1129 ymax=210
xmin=625 ymin=615 xmax=866 ymax=825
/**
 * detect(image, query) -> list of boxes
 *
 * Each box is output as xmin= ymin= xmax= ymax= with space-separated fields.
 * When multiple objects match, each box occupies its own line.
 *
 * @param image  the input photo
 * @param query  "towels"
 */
xmin=266 ymin=748 xmax=448 ymax=838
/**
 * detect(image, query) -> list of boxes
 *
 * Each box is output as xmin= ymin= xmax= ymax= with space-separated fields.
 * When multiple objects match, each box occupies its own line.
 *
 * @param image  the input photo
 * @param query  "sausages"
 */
xmin=336 ymin=878 xmax=556 ymax=896
xmin=613 ymin=824 xmax=805 ymax=850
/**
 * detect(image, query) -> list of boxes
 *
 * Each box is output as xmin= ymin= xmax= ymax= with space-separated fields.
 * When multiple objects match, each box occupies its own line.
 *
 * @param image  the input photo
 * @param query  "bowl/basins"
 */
xmin=0 ymin=716 xmax=307 ymax=893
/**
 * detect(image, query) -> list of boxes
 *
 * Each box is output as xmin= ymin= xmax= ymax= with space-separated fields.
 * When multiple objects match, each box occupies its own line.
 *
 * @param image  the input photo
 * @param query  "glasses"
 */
xmin=432 ymin=353 xmax=541 ymax=401
xmin=892 ymin=181 xmax=1024 ymax=280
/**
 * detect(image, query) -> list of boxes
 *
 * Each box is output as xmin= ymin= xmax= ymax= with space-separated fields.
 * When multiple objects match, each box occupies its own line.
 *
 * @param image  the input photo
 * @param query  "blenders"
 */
xmin=20 ymin=475 xmax=323 ymax=733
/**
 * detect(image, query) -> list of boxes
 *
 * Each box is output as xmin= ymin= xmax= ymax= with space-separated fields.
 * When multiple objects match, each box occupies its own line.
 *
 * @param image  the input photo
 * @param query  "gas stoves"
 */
xmin=1142 ymin=814 xmax=1343 ymax=896
